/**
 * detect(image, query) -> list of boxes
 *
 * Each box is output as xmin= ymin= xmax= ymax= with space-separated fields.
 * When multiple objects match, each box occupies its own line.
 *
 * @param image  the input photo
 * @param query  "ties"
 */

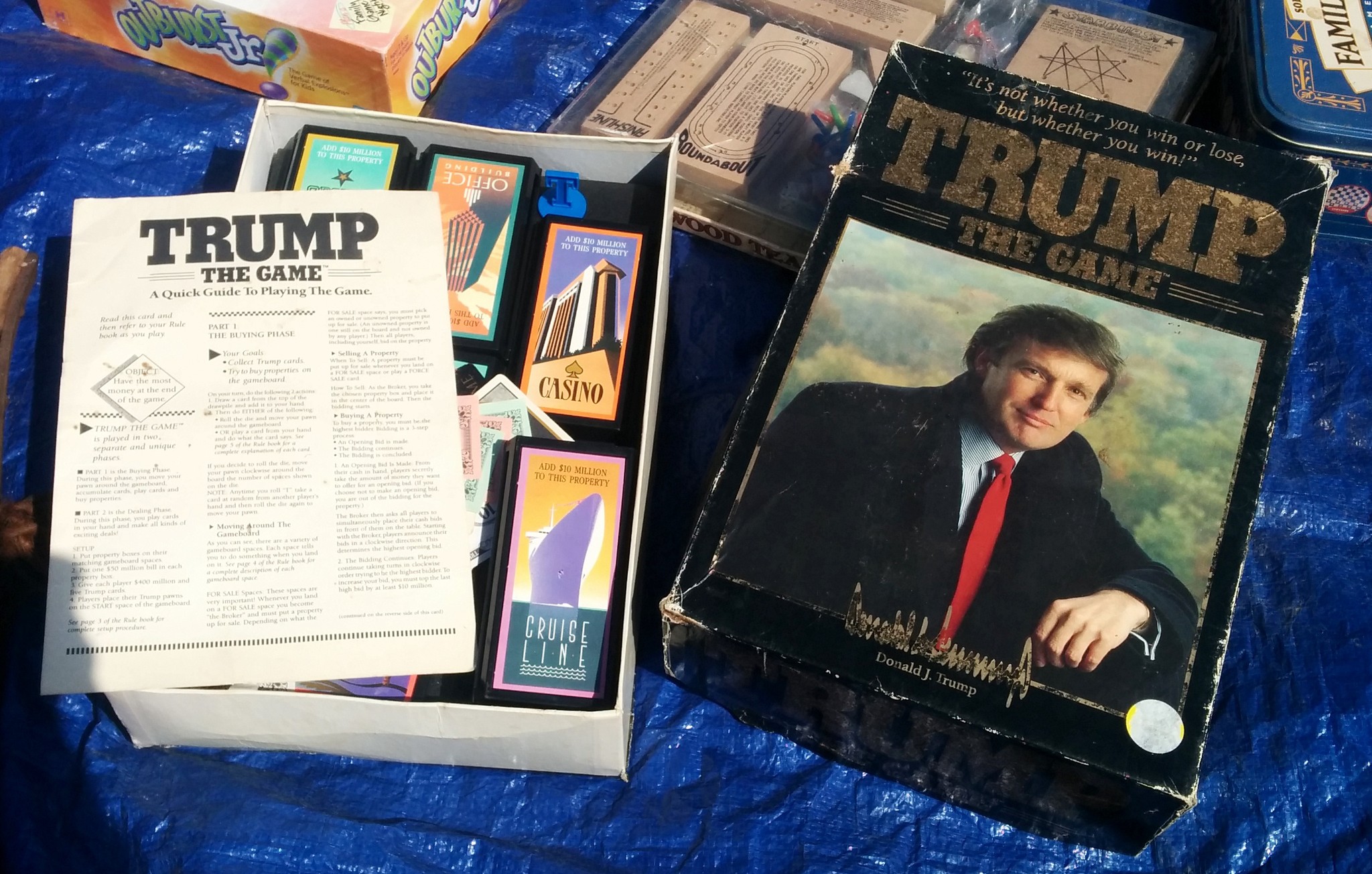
xmin=938 ymin=454 xmax=1018 ymax=651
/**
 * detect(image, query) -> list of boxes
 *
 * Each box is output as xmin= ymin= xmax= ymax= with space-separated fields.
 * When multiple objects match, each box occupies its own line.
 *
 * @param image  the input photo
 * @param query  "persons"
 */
xmin=715 ymin=302 xmax=1201 ymax=745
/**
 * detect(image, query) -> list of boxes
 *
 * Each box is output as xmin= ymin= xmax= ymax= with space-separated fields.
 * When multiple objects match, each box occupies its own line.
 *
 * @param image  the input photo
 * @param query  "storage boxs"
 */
xmin=1229 ymin=1 xmax=1372 ymax=235
xmin=36 ymin=0 xmax=506 ymax=118
xmin=656 ymin=45 xmax=1331 ymax=862
xmin=97 ymin=93 xmax=681 ymax=780
xmin=541 ymin=1 xmax=976 ymax=271
